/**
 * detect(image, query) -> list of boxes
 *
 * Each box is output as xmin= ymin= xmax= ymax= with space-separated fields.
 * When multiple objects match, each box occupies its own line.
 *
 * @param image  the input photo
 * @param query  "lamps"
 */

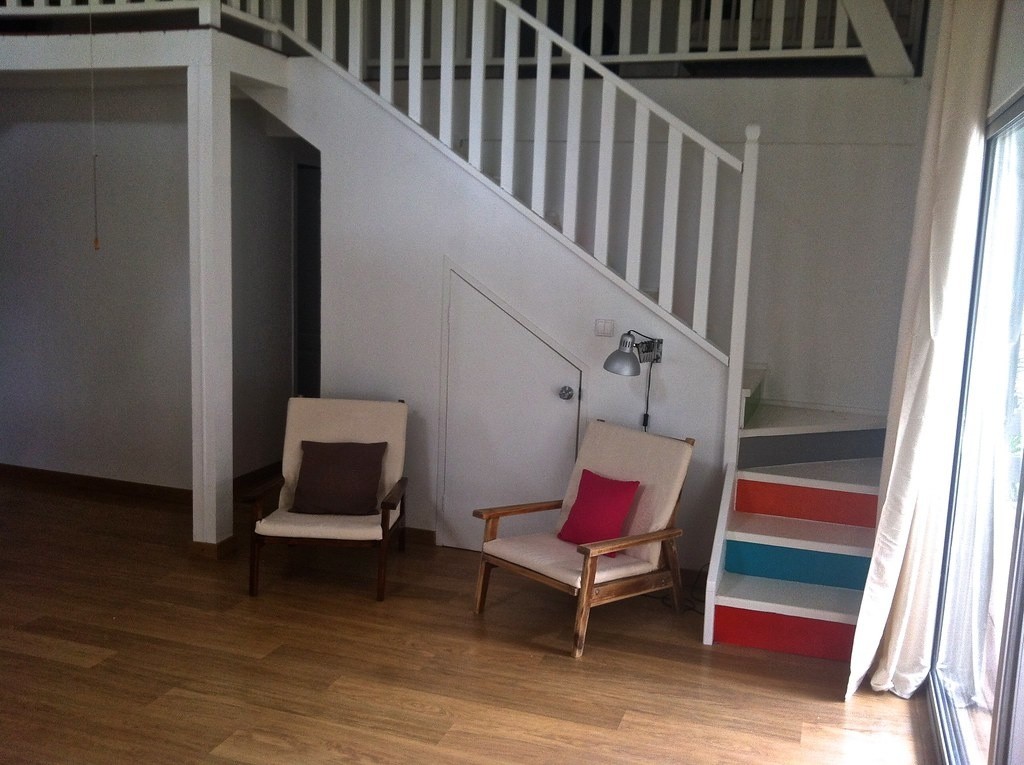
xmin=603 ymin=330 xmax=663 ymax=377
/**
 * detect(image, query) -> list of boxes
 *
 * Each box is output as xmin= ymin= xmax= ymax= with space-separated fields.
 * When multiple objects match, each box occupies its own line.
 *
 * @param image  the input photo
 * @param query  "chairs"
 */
xmin=250 ymin=396 xmax=410 ymax=601
xmin=472 ymin=419 xmax=698 ymax=658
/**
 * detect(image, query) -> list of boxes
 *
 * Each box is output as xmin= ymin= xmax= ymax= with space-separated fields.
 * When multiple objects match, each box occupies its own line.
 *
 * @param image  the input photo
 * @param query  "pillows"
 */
xmin=288 ymin=440 xmax=389 ymax=516
xmin=557 ymin=469 xmax=640 ymax=558
